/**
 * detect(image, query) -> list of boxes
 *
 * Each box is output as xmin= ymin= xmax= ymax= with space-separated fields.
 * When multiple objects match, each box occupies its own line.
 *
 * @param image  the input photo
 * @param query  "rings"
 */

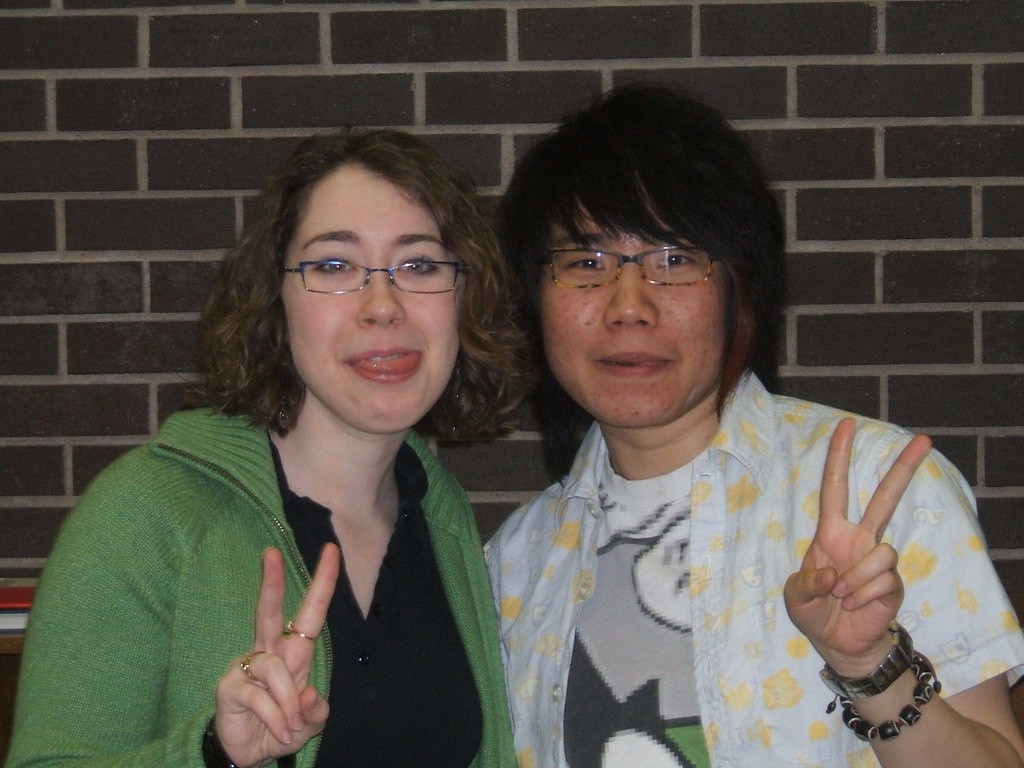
xmin=240 ymin=650 xmax=268 ymax=680
xmin=284 ymin=621 xmax=316 ymax=642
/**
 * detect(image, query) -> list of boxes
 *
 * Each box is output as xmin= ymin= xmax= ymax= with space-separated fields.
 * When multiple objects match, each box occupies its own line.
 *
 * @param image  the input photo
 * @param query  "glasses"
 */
xmin=533 ymin=246 xmax=720 ymax=289
xmin=281 ymin=260 xmax=472 ymax=295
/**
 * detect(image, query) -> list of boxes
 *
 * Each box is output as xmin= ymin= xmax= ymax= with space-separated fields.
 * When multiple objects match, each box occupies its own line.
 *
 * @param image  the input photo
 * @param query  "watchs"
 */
xmin=819 ymin=621 xmax=915 ymax=700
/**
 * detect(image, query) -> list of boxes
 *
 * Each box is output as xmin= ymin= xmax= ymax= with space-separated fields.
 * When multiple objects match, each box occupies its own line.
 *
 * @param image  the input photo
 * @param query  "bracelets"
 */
xmin=826 ymin=651 xmax=942 ymax=741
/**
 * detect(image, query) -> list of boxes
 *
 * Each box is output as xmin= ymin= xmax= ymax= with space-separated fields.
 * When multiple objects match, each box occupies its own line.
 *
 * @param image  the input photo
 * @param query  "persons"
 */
xmin=483 ymin=83 xmax=1024 ymax=768
xmin=4 ymin=126 xmax=534 ymax=768
